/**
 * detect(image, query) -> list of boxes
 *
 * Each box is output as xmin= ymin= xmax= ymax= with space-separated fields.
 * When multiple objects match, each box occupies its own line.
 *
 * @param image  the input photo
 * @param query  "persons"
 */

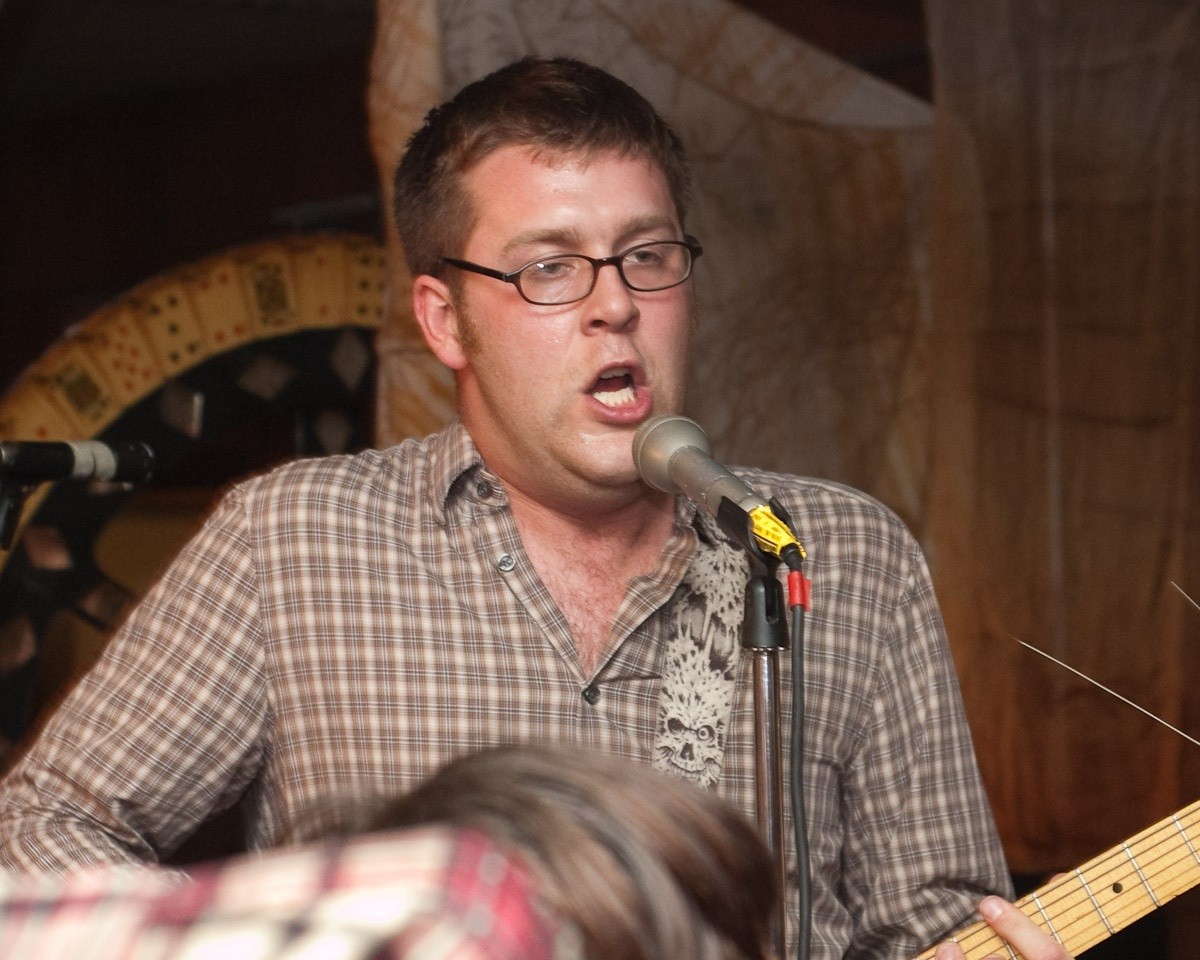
xmin=353 ymin=741 xmax=778 ymax=960
xmin=1 ymin=60 xmax=1069 ymax=960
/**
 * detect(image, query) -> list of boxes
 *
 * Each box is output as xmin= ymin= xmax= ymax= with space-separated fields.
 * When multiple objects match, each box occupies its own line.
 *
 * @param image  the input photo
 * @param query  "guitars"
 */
xmin=904 ymin=800 xmax=1200 ymax=960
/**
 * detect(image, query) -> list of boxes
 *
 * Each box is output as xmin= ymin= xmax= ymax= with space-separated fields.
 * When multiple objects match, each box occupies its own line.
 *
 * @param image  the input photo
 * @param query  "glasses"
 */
xmin=442 ymin=235 xmax=702 ymax=305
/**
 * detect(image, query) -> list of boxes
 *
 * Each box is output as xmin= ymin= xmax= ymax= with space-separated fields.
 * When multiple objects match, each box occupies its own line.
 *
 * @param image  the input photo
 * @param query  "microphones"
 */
xmin=0 ymin=436 xmax=154 ymax=489
xmin=632 ymin=414 xmax=803 ymax=565
